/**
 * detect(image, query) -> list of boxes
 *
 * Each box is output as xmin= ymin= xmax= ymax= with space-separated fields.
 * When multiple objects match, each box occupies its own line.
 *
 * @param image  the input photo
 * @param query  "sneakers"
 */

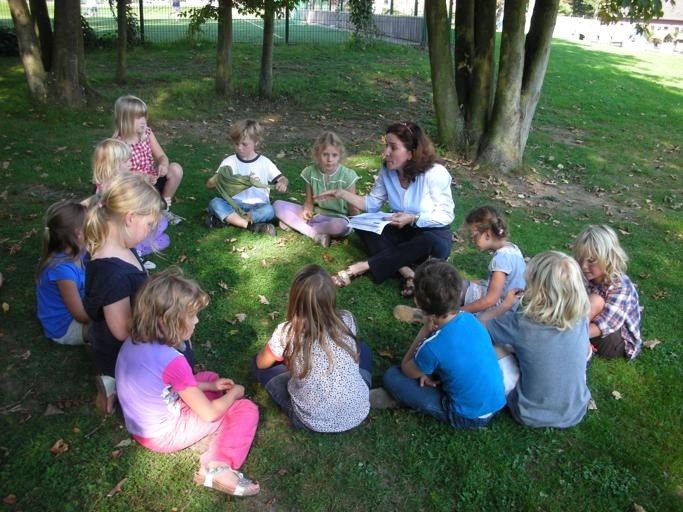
xmin=248 ymin=223 xmax=278 ymax=237
xmin=393 ymin=304 xmax=428 ymax=324
xmin=312 ymin=233 xmax=331 ymax=248
xmin=279 ymin=220 xmax=293 ymax=231
xmin=200 ymin=212 xmax=226 ymax=228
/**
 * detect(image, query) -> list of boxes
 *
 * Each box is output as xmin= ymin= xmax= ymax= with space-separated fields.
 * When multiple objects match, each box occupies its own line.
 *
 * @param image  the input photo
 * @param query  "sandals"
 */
xmin=331 ymin=264 xmax=360 ymax=288
xmin=400 ymin=277 xmax=414 ymax=296
xmin=193 ymin=465 xmax=260 ymax=497
xmin=94 ymin=374 xmax=116 ymax=414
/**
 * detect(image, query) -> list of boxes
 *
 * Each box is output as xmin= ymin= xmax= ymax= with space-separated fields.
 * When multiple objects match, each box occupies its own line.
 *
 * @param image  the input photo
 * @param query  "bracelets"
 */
xmin=410 ymin=214 xmax=414 ymax=227
xmin=414 ymin=215 xmax=419 ymax=223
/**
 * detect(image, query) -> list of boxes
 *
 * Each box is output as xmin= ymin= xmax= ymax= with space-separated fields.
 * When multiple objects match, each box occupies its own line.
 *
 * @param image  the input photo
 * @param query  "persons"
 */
xmin=113 ymin=265 xmax=262 ymax=500
xmin=91 ymin=138 xmax=170 ymax=270
xmin=204 ymin=117 xmax=289 ymax=236
xmin=272 ymin=129 xmax=360 ymax=248
xmin=480 ymin=250 xmax=591 ymax=430
xmin=568 ymin=223 xmax=642 ymax=362
xmin=83 ymin=175 xmax=195 ymax=417
xmin=256 ymin=263 xmax=371 ymax=435
xmin=311 ymin=121 xmax=455 ymax=298
xmin=392 ymin=204 xmax=526 ymax=327
xmin=33 ymin=200 xmax=91 ymax=346
xmin=368 ymin=257 xmax=508 ymax=430
xmin=95 ymin=93 xmax=184 ymax=213
